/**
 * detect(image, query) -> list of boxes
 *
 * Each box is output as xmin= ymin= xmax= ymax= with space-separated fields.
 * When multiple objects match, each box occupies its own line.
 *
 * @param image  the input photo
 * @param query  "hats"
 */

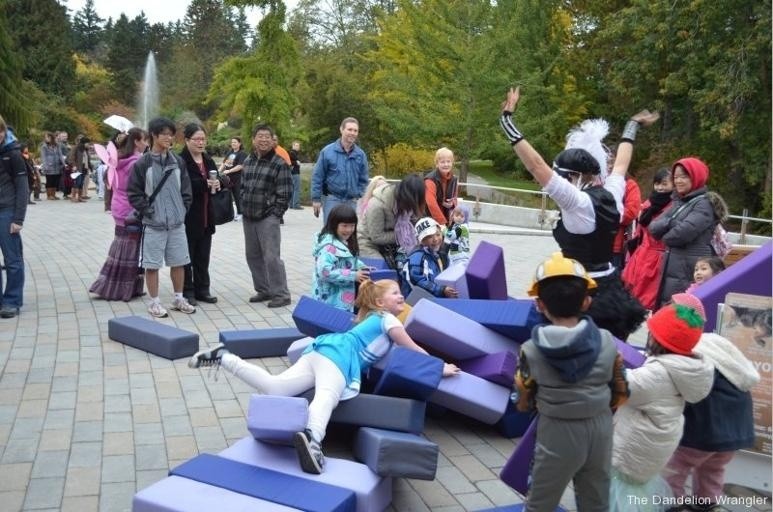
xmin=646 ymin=291 xmax=707 ymax=356
xmin=414 ymin=217 xmax=442 ymax=244
xmin=552 ymin=148 xmax=601 ymax=177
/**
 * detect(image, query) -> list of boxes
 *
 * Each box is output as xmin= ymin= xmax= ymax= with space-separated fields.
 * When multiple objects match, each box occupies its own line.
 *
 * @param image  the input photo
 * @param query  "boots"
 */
xmin=46 ymin=187 xmax=91 ymax=202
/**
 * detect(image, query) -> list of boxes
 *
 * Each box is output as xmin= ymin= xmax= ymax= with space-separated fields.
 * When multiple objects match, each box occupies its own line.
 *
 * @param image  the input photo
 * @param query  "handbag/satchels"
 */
xmin=710 ymin=222 xmax=733 ymax=260
xmin=209 ymin=188 xmax=234 ymax=225
xmin=124 ymin=208 xmax=143 ymax=240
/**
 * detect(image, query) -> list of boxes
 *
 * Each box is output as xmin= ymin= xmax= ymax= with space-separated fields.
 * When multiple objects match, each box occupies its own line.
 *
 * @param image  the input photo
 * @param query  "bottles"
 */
xmin=208 ymin=170 xmax=217 ymax=194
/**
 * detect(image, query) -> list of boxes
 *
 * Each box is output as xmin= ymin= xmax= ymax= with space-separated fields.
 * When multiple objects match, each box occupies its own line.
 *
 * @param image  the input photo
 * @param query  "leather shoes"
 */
xmin=268 ymin=297 xmax=290 ymax=308
xmin=249 ymin=293 xmax=272 ymax=302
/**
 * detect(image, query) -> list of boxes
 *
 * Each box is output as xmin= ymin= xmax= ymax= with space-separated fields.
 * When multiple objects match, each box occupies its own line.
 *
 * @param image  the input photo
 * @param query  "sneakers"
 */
xmin=147 ymin=301 xmax=168 ymax=317
xmin=29 ymin=198 xmax=41 ymax=204
xmin=294 ymin=428 xmax=326 ymax=474
xmin=170 ymin=295 xmax=216 ymax=314
xmin=0 ymin=304 xmax=20 ymax=318
xmin=188 ymin=342 xmax=230 ymax=381
xmin=663 ymin=494 xmax=719 ymax=511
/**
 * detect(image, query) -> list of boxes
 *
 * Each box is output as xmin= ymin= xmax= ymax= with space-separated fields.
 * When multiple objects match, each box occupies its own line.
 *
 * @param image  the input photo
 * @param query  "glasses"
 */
xmin=188 ymin=136 xmax=207 ymax=143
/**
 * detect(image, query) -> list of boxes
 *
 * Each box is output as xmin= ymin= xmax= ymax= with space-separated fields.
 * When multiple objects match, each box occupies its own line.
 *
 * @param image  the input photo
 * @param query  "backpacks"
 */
xmin=2 ymin=144 xmax=36 ymax=191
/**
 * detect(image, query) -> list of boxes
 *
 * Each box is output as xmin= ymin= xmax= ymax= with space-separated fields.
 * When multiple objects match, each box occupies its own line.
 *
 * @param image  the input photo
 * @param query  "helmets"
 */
xmin=527 ymin=252 xmax=598 ymax=297
